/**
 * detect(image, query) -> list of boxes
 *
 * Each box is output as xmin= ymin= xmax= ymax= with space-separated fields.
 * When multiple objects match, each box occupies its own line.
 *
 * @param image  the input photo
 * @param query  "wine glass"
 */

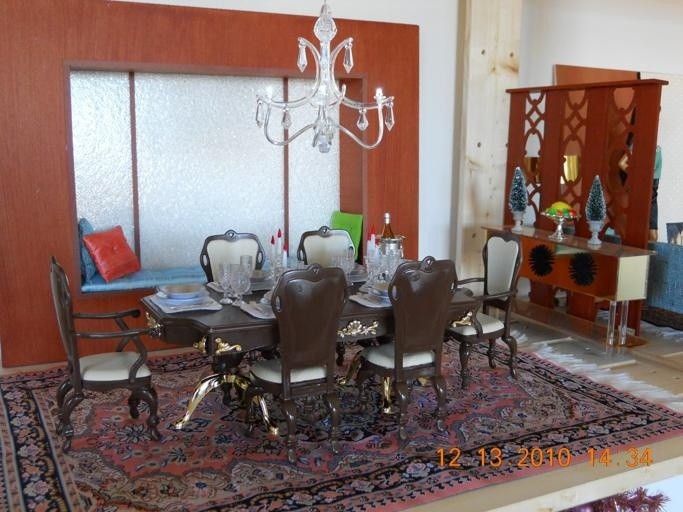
xmin=218 ymin=248 xmax=412 ymax=307
xmin=586 ymin=221 xmax=605 ymax=245
xmin=540 ymin=212 xmax=581 ymax=240
xmin=510 ymin=210 xmax=526 ymax=232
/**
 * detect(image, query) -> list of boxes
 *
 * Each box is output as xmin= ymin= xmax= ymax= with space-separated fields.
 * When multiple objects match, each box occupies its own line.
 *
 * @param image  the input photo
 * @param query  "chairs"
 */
xmin=49 ymin=255 xmax=160 ymax=455
xmin=297 ymin=224 xmax=356 ymax=270
xmin=446 ymin=230 xmax=523 ymax=390
xmin=200 ymin=230 xmax=267 ymax=282
xmin=355 ymin=255 xmax=458 ymax=440
xmin=243 ymin=263 xmax=348 ymax=464
xmin=640 ymin=241 xmax=682 ymax=332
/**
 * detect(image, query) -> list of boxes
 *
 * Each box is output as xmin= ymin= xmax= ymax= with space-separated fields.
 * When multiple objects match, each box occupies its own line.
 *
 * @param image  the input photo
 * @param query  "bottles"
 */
xmin=382 ymin=213 xmax=394 ymax=239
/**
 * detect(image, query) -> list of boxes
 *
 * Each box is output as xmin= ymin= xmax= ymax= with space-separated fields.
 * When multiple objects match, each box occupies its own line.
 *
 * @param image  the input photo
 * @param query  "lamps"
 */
xmin=523 ymin=152 xmax=579 ymax=185
xmin=253 ymin=0 xmax=396 ymax=156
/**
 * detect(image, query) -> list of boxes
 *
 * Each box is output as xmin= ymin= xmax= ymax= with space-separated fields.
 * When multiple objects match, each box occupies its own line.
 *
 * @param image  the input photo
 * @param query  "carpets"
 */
xmin=0 ymin=326 xmax=682 ymax=511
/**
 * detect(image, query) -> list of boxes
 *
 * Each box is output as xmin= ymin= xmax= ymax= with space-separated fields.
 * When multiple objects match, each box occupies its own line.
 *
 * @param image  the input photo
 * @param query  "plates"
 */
xmin=205 ymin=282 xmax=223 ymax=293
xmin=149 ymin=293 xmax=222 ymax=314
xmin=238 ymin=303 xmax=276 ymax=320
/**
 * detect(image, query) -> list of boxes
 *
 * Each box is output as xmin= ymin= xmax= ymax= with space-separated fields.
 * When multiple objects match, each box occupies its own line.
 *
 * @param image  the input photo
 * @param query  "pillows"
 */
xmin=79 ymin=218 xmax=96 ymax=281
xmin=81 ymin=225 xmax=142 ymax=285
xmin=328 ymin=210 xmax=363 ymax=262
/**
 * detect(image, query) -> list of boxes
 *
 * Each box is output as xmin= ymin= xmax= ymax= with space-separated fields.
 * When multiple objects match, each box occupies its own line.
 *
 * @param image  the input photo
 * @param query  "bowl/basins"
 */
xmin=158 ymin=285 xmax=202 ymax=299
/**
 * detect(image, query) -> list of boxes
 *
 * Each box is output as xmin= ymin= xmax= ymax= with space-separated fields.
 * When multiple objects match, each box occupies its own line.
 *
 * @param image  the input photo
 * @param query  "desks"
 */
xmin=476 ymin=222 xmax=656 ymax=350
